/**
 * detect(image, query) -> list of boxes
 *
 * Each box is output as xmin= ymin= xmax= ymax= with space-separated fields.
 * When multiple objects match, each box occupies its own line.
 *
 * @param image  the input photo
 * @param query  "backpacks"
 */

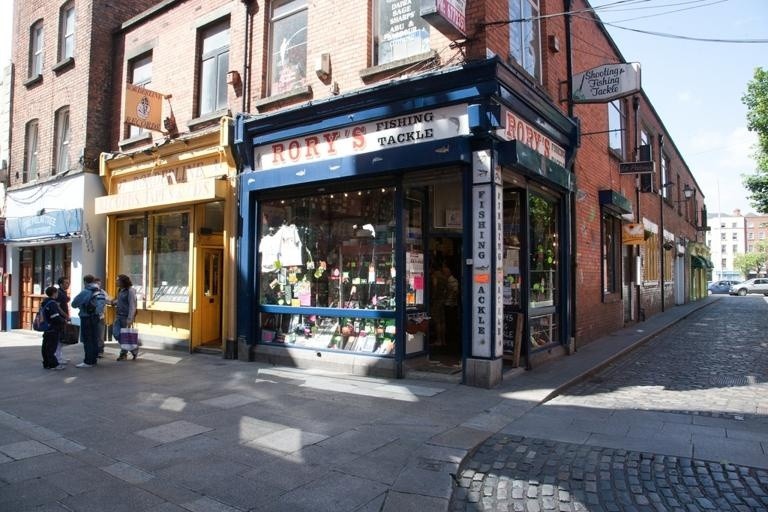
xmin=32 ymin=299 xmax=57 ymax=332
xmin=86 ymin=286 xmax=107 ymax=316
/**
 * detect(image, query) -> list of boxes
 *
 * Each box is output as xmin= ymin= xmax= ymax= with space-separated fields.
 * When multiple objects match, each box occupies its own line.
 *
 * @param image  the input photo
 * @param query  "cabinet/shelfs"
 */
xmin=339 ymin=244 xmax=395 ymax=311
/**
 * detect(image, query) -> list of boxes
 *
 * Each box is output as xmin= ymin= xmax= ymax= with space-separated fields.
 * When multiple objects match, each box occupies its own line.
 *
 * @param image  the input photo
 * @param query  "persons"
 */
xmin=92 ymin=278 xmax=111 ymax=359
xmin=259 ymin=227 xmax=281 ymax=273
xmin=71 ymin=274 xmax=106 ymax=368
xmin=53 ymin=276 xmax=71 ymax=365
xmin=271 ymin=223 xmax=303 ymax=267
xmin=105 ymin=273 xmax=143 ymax=361
xmin=41 ymin=286 xmax=70 ymax=370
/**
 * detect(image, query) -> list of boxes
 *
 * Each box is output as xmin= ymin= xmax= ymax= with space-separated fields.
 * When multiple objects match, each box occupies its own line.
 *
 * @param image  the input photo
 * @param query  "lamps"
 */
xmin=674 ymin=184 xmax=694 ymax=202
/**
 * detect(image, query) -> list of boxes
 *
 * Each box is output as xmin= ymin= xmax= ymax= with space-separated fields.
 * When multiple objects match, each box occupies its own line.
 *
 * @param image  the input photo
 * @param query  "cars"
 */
xmin=708 ymin=281 xmax=740 ymax=294
xmin=730 ymin=277 xmax=768 ymax=295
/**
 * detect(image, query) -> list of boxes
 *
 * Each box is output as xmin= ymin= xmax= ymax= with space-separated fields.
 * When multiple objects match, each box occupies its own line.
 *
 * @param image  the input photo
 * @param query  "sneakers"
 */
xmin=47 ymin=351 xmax=139 ymax=370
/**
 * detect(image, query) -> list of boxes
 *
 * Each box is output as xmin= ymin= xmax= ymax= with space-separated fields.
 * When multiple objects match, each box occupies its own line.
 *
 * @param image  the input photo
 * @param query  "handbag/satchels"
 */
xmin=119 ymin=323 xmax=140 ymax=351
